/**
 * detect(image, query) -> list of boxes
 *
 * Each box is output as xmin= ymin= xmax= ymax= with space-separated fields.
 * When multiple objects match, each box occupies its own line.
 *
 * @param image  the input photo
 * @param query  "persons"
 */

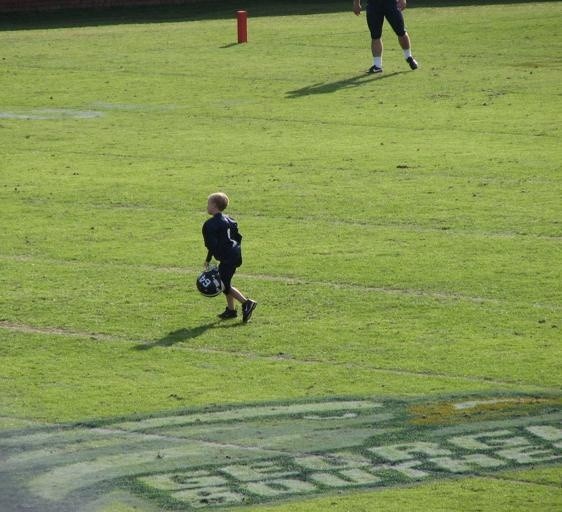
xmin=201 ymin=192 xmax=257 ymax=323
xmin=352 ymin=0 xmax=418 ymax=73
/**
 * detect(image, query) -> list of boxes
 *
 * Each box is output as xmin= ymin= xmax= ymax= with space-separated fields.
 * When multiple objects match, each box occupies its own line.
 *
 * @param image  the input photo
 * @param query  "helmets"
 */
xmin=196 ymin=270 xmax=226 ymax=298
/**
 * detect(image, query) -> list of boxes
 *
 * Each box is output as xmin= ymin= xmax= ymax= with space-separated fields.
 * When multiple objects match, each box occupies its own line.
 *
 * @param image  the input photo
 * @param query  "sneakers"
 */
xmin=368 ymin=65 xmax=382 ymax=73
xmin=407 ymin=57 xmax=417 ymax=69
xmin=242 ymin=299 xmax=256 ymax=322
xmin=219 ymin=306 xmax=237 ymax=318
